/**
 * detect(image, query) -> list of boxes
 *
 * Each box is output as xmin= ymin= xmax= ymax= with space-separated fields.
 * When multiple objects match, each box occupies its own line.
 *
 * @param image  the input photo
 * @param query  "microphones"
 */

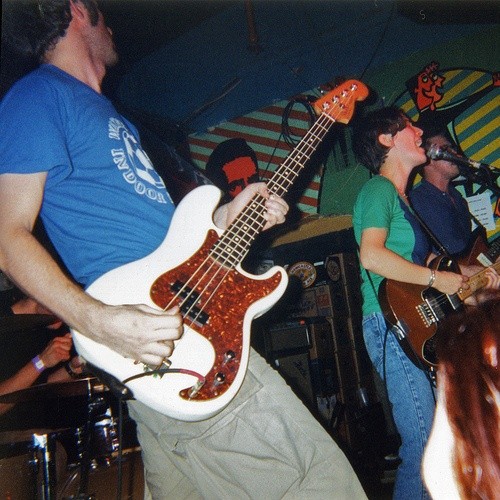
xmin=426 ymin=145 xmax=481 ymax=167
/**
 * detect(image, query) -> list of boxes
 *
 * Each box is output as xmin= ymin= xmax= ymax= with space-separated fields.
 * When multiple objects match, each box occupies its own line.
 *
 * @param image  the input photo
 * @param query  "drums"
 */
xmin=87 ymin=415 xmax=143 ymax=464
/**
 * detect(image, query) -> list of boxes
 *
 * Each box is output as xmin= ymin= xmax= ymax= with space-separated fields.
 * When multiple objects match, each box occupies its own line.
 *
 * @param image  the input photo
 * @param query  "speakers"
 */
xmin=251 ymin=320 xmax=349 ymax=448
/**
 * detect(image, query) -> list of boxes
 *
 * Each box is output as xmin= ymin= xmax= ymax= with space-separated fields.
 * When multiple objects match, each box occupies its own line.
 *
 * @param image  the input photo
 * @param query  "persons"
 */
xmin=0 ymin=0 xmax=368 ymax=500
xmin=0 ymin=286 xmax=85 ymax=431
xmin=352 ymin=105 xmax=500 ymax=500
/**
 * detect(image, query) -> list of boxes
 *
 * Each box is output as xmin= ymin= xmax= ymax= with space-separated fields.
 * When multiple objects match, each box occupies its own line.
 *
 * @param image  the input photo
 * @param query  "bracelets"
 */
xmin=428 ymin=268 xmax=435 ymax=286
xmin=65 ymin=363 xmax=79 ymax=377
xmin=32 ymin=355 xmax=45 ymax=373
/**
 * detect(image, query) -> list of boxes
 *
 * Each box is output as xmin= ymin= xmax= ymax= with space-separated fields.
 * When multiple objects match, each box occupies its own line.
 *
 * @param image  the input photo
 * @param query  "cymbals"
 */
xmin=0 ymin=376 xmax=116 ymax=404
xmin=0 ymin=313 xmax=62 ymax=336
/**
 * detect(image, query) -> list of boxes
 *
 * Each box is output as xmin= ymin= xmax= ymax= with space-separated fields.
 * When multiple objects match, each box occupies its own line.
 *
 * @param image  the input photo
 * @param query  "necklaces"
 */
xmin=381 ymin=174 xmax=408 ymax=198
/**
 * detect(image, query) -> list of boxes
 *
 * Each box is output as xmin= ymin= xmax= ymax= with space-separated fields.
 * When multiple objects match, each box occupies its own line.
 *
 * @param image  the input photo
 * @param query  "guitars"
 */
xmin=69 ymin=77 xmax=371 ymax=422
xmin=377 ymin=225 xmax=500 ymax=370
